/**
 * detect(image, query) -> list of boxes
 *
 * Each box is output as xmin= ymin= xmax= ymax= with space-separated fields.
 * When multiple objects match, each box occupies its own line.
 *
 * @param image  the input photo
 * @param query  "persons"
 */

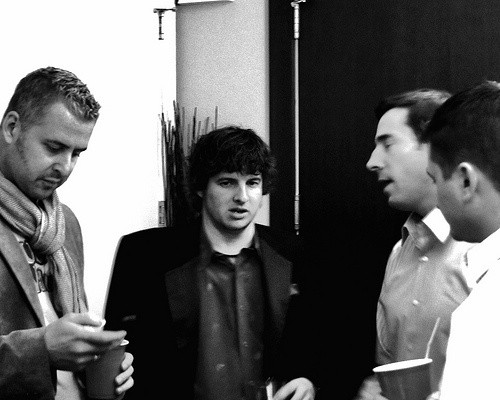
xmin=426 ymin=77 xmax=499 ymax=400
xmin=0 ymin=67 xmax=135 ymax=400
xmin=352 ymin=87 xmax=479 ymax=400
xmin=103 ymin=126 xmax=336 ymax=399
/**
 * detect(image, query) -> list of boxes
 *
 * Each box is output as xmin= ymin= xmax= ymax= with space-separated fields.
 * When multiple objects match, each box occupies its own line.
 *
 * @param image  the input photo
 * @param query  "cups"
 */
xmin=72 ymin=340 xmax=129 ymax=400
xmin=372 ymin=358 xmax=433 ymax=400
